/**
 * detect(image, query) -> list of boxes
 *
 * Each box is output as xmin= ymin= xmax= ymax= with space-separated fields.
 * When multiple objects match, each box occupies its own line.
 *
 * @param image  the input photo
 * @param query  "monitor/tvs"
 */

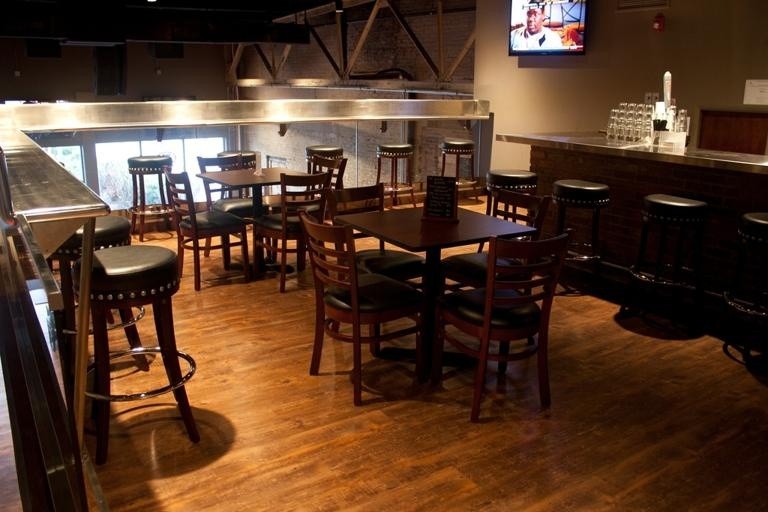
xmin=508 ymin=0 xmax=587 ymax=56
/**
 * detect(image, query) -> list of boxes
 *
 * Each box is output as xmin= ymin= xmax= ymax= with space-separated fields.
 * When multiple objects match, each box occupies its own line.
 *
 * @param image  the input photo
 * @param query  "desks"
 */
xmin=334 ymin=204 xmax=537 ymax=384
xmin=1 ymin=129 xmax=110 ymax=469
xmin=196 ymin=167 xmax=312 ymax=277
xmin=0 ymin=100 xmax=491 ymax=215
xmin=496 ymin=130 xmax=768 ymax=336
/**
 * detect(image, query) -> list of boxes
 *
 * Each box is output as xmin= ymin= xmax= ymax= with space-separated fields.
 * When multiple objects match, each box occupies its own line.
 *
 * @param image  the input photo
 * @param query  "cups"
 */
xmin=619 ymin=103 xmax=627 ymax=110
xmin=678 ymin=109 xmax=687 ymax=117
xmin=646 ymin=105 xmax=654 ymax=113
xmin=626 ymin=112 xmax=634 ymax=119
xmin=607 ymin=123 xmax=617 ymax=145
xmin=652 ymin=93 xmax=659 ymax=105
xmin=634 ymin=126 xmax=641 ymax=145
xmin=617 ymin=125 xmax=625 ymax=146
xmin=611 ymin=109 xmax=619 ymax=117
xmin=643 ymin=113 xmax=651 ymax=122
xmin=643 ymin=127 xmax=651 ymax=138
xmin=625 ymin=120 xmax=633 ymax=125
xmin=617 ymin=119 xmax=625 ymax=125
xmin=644 ymin=92 xmax=651 ymax=105
xmin=678 ymin=119 xmax=687 ymax=131
xmin=637 ymin=104 xmax=645 ymax=112
xmin=626 ymin=126 xmax=633 ymax=146
xmin=608 ymin=117 xmax=616 ymax=123
xmin=642 ymin=122 xmax=651 ymax=127
xmin=619 ymin=110 xmax=626 ymax=118
xmin=634 ymin=121 xmax=642 ymax=126
xmin=635 ymin=112 xmax=642 ymax=121
xmin=627 ymin=104 xmax=637 ymax=111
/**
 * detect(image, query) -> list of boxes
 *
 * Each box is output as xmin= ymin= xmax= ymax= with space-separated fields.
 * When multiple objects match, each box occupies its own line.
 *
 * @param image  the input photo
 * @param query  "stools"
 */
xmin=618 ymin=193 xmax=709 ymax=325
xmin=72 ymin=244 xmax=206 ymax=464
xmin=486 ymin=167 xmax=540 ymax=236
xmin=442 ymin=138 xmax=480 ymax=205
xmin=306 ymin=145 xmax=344 ymax=212
xmin=128 ymin=156 xmax=176 ymax=241
xmin=377 ymin=144 xmax=417 ymax=207
xmin=724 ymin=213 xmax=768 ymax=360
xmin=552 ymin=179 xmax=610 ymax=293
xmin=217 ymin=152 xmax=262 ymax=197
xmin=62 ymin=216 xmax=152 ymax=371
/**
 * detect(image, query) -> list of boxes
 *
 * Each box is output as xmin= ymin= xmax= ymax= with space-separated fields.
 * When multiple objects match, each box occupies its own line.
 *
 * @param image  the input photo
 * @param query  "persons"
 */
xmin=511 ymin=0 xmax=564 ymax=50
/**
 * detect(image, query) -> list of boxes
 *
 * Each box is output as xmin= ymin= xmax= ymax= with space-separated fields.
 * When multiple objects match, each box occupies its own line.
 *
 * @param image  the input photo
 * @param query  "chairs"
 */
xmin=255 ymin=172 xmax=335 ymax=293
xmin=443 ymin=191 xmax=552 ymax=293
xmin=323 ymin=183 xmax=428 ymax=285
xmin=196 ymin=155 xmax=269 ymax=257
xmin=442 ymin=236 xmax=566 ymax=421
xmin=162 ymin=167 xmax=248 ymax=291
xmin=295 ymin=211 xmax=433 ymax=406
xmin=309 ymin=155 xmax=348 ymax=190
